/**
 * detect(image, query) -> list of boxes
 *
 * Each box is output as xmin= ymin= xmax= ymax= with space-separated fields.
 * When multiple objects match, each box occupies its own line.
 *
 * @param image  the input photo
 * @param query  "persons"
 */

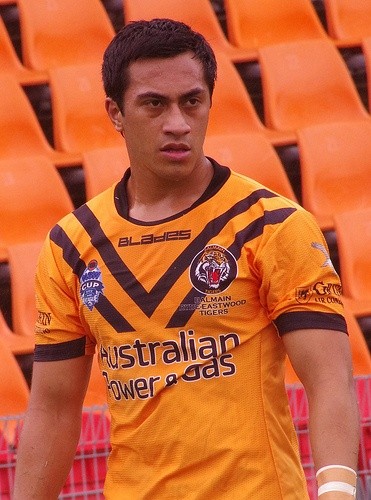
xmin=5 ymin=18 xmax=364 ymax=500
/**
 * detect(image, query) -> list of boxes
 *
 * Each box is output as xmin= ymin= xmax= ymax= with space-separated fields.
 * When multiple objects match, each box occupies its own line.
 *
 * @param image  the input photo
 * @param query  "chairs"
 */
xmin=333 ymin=207 xmax=371 ymax=316
xmin=0 ymin=16 xmax=48 ymax=84
xmin=125 ymin=0 xmax=259 ymax=62
xmin=48 ymin=64 xmax=125 ymax=153
xmin=202 ymin=132 xmax=300 ymax=203
xmin=17 ymin=0 xmax=117 ymax=72
xmin=325 ymin=0 xmax=371 ymax=114
xmin=225 ymin=0 xmax=332 ymax=49
xmin=256 ymin=38 xmax=370 ymax=130
xmin=297 ymin=117 xmax=371 ymax=230
xmin=0 ymin=73 xmax=81 ymax=168
xmin=0 ymin=154 xmax=76 ymax=261
xmin=81 ymin=147 xmax=130 ymax=201
xmin=205 ymin=54 xmax=268 ymax=135
xmin=0 ymin=242 xmax=108 ymax=417
